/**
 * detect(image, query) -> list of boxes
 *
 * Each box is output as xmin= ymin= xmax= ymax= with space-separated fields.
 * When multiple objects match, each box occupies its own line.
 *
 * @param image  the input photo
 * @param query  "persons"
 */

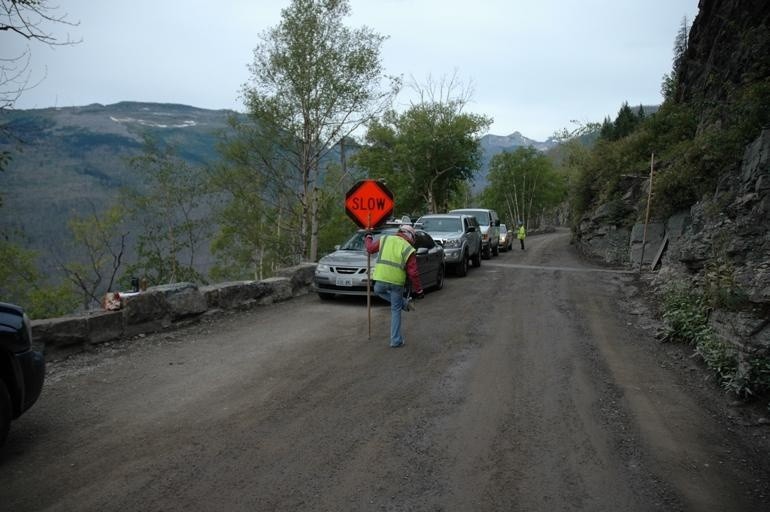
xmin=364 ymin=224 xmax=425 ymax=347
xmin=516 ymin=221 xmax=526 ymax=249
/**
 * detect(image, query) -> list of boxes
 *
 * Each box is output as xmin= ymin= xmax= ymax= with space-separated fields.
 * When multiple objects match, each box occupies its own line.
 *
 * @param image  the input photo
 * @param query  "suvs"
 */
xmin=413 ymin=213 xmax=482 ymax=276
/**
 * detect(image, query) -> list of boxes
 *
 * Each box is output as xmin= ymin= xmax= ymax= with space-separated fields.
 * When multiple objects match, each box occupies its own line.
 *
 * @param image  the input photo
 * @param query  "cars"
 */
xmin=498 ymin=223 xmax=514 ymax=251
xmin=0 ymin=298 xmax=46 ymax=449
xmin=310 ymin=216 xmax=446 ymax=304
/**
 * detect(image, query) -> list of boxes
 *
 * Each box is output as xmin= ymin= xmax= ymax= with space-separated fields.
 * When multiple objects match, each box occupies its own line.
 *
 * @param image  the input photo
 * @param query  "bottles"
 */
xmin=141 ymin=277 xmax=147 ymax=291
xmin=132 ymin=277 xmax=139 ymax=292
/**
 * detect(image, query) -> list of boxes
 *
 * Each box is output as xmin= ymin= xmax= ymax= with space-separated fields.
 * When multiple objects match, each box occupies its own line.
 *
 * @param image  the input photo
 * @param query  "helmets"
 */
xmin=396 ymin=224 xmax=416 ymax=246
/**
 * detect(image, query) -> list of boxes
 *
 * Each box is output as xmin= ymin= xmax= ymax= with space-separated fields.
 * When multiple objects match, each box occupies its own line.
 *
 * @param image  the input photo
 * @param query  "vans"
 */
xmin=446 ymin=209 xmax=502 ymax=259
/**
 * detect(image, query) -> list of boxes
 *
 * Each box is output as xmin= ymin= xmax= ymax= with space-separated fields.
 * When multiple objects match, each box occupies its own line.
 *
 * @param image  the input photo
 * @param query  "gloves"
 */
xmin=365 ymin=230 xmax=372 ymax=238
xmin=416 ymin=290 xmax=424 ymax=299
